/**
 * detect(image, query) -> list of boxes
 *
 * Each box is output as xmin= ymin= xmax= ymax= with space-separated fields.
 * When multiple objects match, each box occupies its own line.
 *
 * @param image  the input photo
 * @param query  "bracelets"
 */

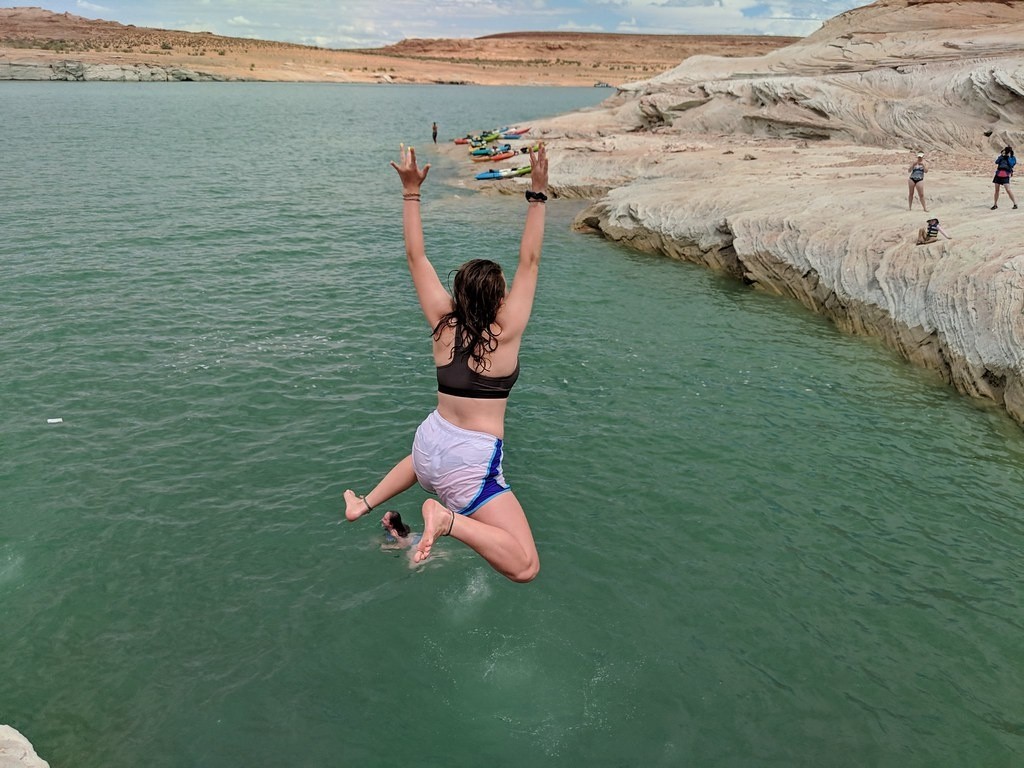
xmin=403 ymin=193 xmax=421 ymax=202
xmin=526 ymin=190 xmax=548 ymax=204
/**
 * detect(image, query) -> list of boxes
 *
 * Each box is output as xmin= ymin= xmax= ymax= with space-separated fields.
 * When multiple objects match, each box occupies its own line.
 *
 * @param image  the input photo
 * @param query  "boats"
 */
xmin=453 ymin=126 xmax=533 ymax=162
xmin=474 ymin=166 xmax=532 ymax=180
xmin=520 ymin=140 xmax=549 ymax=155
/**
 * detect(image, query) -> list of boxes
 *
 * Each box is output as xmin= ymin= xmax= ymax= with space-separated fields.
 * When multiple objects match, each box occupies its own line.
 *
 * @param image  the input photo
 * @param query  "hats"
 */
xmin=926 ymin=215 xmax=939 ymax=224
xmin=917 ymin=152 xmax=924 ymax=157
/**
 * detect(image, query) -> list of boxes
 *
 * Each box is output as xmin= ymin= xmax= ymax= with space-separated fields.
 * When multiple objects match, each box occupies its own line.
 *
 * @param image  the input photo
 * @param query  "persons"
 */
xmin=380 ymin=510 xmax=402 ymax=537
xmin=344 ymin=143 xmax=549 ymax=581
xmin=907 ymin=151 xmax=931 ymax=210
xmin=917 ymin=217 xmax=952 ymax=244
xmin=991 ymin=147 xmax=1017 ymax=210
xmin=432 ymin=122 xmax=437 ymax=142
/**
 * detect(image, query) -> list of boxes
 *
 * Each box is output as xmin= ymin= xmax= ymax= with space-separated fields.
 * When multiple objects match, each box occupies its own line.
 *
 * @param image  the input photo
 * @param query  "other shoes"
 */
xmin=991 ymin=205 xmax=997 ymax=210
xmin=1013 ymin=205 xmax=1017 ymax=209
